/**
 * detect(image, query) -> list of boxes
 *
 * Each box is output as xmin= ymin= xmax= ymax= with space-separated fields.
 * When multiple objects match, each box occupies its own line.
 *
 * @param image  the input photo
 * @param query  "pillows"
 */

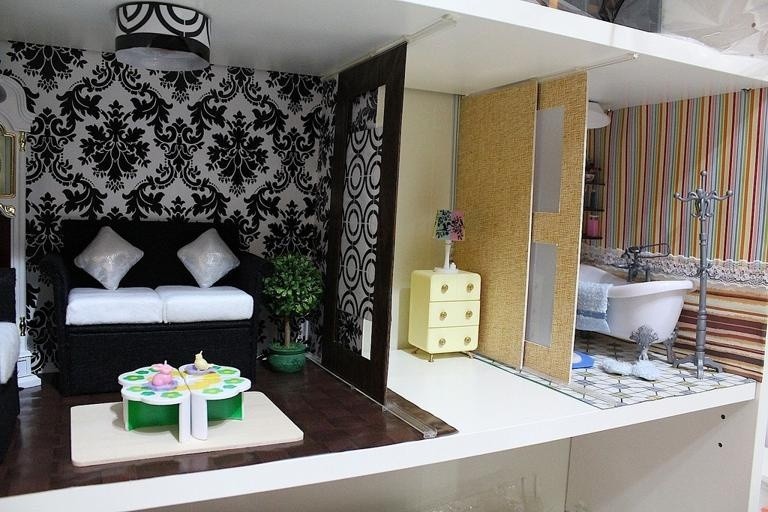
xmin=70 ymin=227 xmax=146 ymax=290
xmin=173 ymin=225 xmax=239 ymax=288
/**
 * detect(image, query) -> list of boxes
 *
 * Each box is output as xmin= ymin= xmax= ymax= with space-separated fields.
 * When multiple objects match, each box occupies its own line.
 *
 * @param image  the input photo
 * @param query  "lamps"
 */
xmin=586 ymin=101 xmax=611 ymax=132
xmin=115 ymin=3 xmax=211 ymax=71
xmin=431 ymin=209 xmax=467 ymax=273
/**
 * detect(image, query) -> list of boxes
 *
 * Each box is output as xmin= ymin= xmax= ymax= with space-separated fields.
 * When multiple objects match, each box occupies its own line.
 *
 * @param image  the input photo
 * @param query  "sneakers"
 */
xmin=601 ymin=355 xmax=658 ymax=382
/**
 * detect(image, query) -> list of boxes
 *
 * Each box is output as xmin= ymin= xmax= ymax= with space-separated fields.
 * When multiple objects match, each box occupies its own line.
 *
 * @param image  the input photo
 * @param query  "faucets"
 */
xmin=620 ymin=248 xmax=631 ymax=270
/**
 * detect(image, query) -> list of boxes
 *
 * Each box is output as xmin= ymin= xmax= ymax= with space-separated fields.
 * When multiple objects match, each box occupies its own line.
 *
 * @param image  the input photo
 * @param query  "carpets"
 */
xmin=466 ymin=335 xmax=754 ymax=408
xmin=70 ymin=390 xmax=304 ymax=467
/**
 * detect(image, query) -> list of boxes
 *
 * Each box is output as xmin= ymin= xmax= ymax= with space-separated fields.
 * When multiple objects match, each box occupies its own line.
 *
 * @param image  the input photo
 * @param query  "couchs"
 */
xmin=44 ymin=216 xmax=274 ymax=394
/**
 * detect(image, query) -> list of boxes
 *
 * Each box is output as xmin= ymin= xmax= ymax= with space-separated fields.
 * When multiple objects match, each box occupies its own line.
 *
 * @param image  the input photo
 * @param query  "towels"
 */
xmin=575 ymin=282 xmax=613 ymax=333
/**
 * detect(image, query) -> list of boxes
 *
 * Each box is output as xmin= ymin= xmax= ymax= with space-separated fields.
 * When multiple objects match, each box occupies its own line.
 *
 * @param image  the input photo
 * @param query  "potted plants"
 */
xmin=586 ymin=158 xmax=602 ymax=182
xmin=259 ymin=252 xmax=322 ymax=373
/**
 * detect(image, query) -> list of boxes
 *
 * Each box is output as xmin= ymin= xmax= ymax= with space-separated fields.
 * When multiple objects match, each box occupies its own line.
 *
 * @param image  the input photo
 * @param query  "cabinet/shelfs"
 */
xmin=582 ymin=180 xmax=604 ymax=240
xmin=409 ymin=266 xmax=480 ymax=362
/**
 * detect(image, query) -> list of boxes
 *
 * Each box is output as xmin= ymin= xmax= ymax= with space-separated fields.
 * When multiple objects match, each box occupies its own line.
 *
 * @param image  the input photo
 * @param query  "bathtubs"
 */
xmin=576 ymin=264 xmax=693 ymax=365
